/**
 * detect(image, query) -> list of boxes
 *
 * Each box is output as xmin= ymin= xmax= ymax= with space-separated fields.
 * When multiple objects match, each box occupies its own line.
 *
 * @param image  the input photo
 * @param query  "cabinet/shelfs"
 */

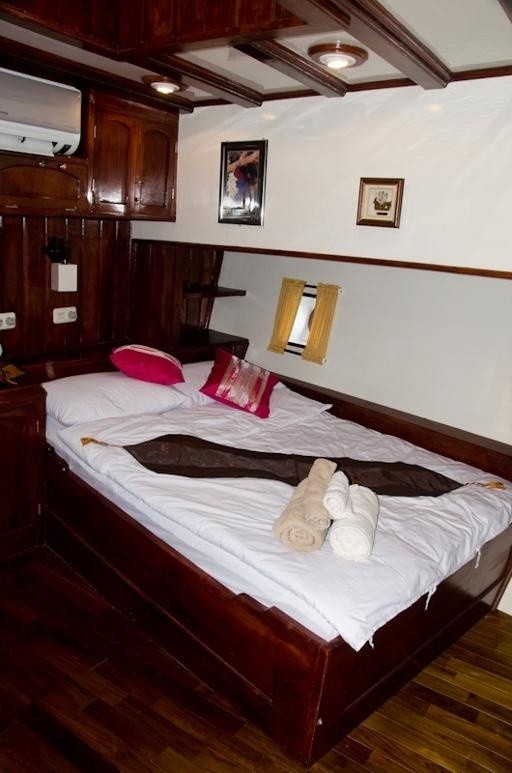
xmin=90 ymin=113 xmax=177 ymax=221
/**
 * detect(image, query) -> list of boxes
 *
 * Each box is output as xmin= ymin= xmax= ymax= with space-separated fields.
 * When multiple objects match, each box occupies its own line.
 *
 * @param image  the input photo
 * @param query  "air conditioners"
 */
xmin=1 ymin=64 xmax=82 ymax=158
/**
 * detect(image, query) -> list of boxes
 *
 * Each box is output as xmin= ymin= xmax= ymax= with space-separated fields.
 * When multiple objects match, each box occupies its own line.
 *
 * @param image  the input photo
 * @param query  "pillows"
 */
xmin=199 ymin=347 xmax=280 ymax=419
xmin=164 ymin=360 xmax=286 ymax=408
xmin=41 ymin=372 xmax=189 ymax=428
xmin=111 ymin=344 xmax=186 ymax=385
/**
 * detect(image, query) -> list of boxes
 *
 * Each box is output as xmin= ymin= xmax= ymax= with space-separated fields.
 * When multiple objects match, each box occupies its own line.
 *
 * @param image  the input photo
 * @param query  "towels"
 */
xmin=304 ymin=458 xmax=334 ymax=527
xmin=325 ymin=472 xmax=351 ymax=516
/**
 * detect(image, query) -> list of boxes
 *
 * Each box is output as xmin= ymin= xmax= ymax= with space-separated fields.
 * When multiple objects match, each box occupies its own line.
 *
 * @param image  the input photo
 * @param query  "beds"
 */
xmin=38 ymin=360 xmax=512 ymax=768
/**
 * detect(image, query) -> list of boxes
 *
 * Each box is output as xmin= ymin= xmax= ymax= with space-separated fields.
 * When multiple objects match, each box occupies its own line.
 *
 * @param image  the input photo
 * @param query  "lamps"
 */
xmin=309 ymin=43 xmax=370 ymax=75
xmin=141 ymin=71 xmax=191 ymax=97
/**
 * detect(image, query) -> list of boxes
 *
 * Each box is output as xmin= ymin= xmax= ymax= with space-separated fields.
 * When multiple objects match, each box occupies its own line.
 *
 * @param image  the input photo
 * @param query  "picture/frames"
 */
xmin=215 ymin=140 xmax=268 ymax=229
xmin=354 ymin=177 xmax=404 ymax=228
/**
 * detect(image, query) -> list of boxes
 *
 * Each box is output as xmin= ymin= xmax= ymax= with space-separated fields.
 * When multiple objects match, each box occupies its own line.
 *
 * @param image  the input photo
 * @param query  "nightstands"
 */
xmin=172 ymin=327 xmax=249 ymax=362
